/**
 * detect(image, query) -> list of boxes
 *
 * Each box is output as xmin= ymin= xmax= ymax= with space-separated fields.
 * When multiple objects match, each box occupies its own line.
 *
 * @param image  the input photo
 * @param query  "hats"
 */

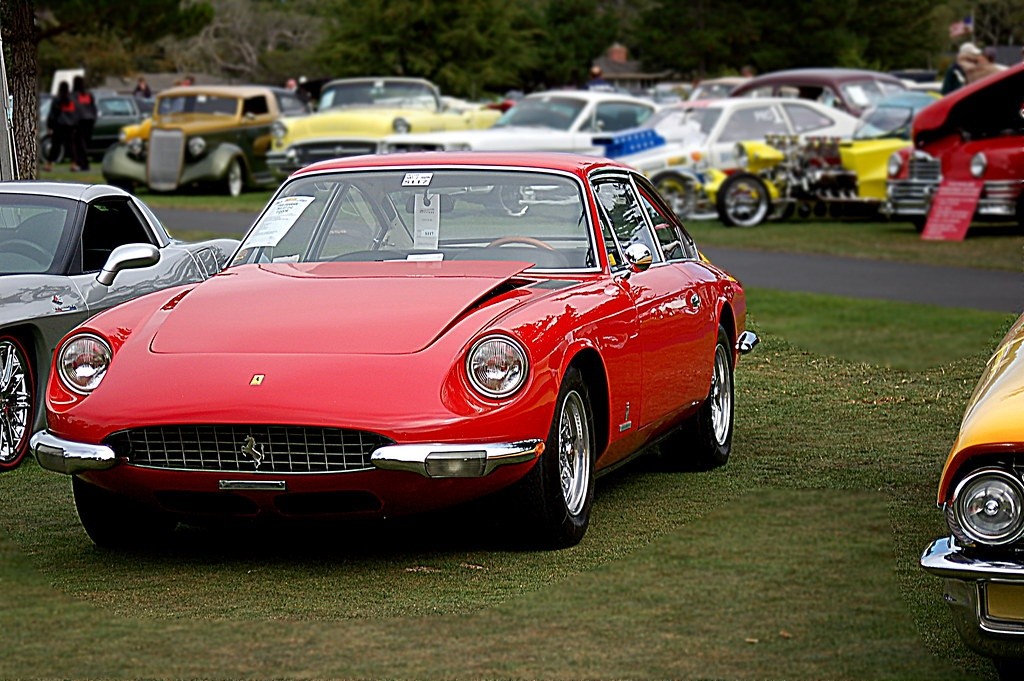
xmin=957 ymin=43 xmax=982 ymax=56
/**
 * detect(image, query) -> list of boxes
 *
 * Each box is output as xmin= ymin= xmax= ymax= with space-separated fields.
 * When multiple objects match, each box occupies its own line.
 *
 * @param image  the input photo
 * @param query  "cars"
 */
xmin=0 ymin=179 xmax=274 ymax=472
xmin=101 ymin=84 xmax=315 ymax=198
xmin=918 ymin=310 xmax=1023 ymax=681
xmin=29 ymin=149 xmax=759 ymax=553
xmin=34 ymin=67 xmax=944 ymax=230
xmin=879 ymin=60 xmax=1024 ymax=230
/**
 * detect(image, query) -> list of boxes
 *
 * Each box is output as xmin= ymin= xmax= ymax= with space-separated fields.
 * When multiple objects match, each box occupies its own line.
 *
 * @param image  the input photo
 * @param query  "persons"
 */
xmin=43 ymin=73 xmax=298 ymax=174
xmin=942 ymin=44 xmax=997 ymax=94
xmin=742 ymin=65 xmax=758 ymax=96
xmin=584 ymin=66 xmax=615 ymax=91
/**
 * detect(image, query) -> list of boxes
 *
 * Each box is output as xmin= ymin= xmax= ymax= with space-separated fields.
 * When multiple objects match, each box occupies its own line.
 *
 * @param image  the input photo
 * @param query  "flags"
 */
xmin=949 ymin=17 xmax=973 ymax=37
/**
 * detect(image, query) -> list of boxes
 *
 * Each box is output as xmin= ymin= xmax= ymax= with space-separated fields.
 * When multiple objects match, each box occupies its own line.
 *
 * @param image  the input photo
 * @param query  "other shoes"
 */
xmin=42 ymin=164 xmax=52 ymax=171
xmin=70 ymin=163 xmax=78 ymax=171
